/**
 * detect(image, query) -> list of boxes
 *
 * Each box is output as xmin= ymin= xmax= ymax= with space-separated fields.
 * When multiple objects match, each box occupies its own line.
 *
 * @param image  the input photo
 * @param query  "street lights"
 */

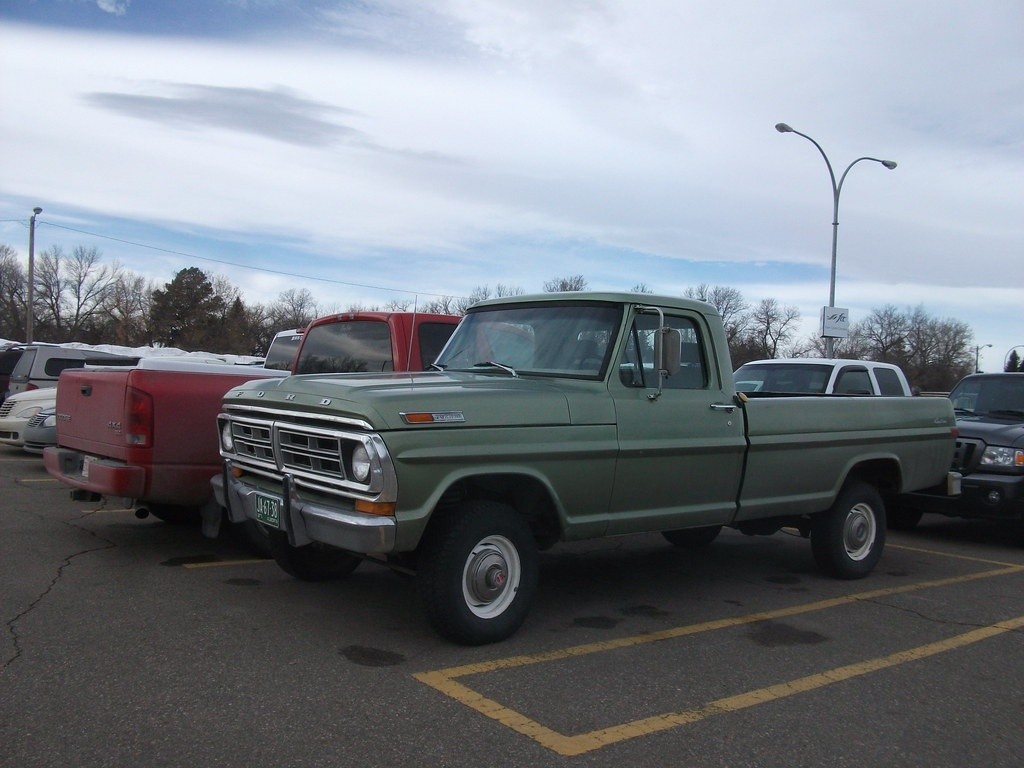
xmin=26 ymin=205 xmax=45 ymax=343
xmin=776 ymin=121 xmax=897 ymax=359
xmin=974 ymin=343 xmax=993 ymax=373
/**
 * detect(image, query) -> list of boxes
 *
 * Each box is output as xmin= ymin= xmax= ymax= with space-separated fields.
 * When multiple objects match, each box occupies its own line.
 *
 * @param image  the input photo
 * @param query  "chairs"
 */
xmin=648 ymin=342 xmax=703 ymax=389
xmin=562 ymin=338 xmax=596 ymax=369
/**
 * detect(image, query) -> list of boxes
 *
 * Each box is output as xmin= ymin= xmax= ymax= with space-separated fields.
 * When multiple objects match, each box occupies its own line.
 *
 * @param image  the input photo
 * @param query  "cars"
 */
xmin=732 ymin=356 xmax=1024 ymax=530
xmin=0 ymin=327 xmax=345 ymax=455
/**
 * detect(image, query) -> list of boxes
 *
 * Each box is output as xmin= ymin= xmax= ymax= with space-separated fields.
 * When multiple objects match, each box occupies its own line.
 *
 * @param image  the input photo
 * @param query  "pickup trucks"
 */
xmin=208 ymin=290 xmax=958 ymax=646
xmin=43 ymin=311 xmax=533 ymax=526
